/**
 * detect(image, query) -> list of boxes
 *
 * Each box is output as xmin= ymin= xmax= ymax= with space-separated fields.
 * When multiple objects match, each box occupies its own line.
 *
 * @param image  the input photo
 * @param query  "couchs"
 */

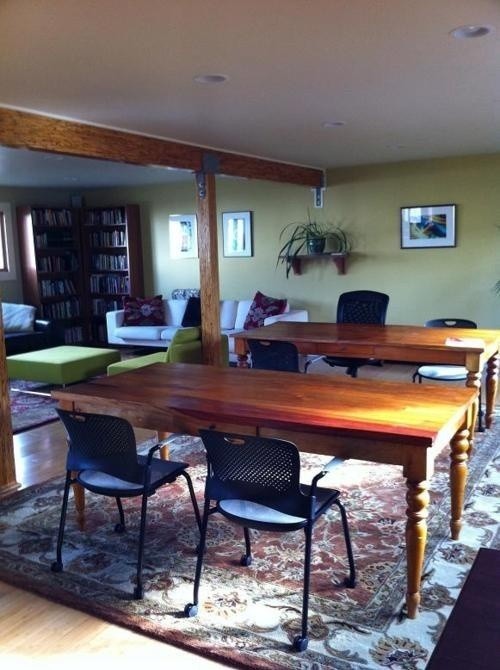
xmin=105 ymin=298 xmax=311 ymax=354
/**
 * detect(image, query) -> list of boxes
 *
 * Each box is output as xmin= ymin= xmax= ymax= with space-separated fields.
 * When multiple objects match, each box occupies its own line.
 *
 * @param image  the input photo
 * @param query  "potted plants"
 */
xmin=277 ymin=208 xmax=355 ymax=281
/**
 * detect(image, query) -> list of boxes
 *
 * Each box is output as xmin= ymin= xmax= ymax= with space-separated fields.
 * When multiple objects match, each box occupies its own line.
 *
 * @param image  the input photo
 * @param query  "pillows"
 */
xmin=180 ymin=296 xmax=204 ymax=327
xmin=120 ymin=293 xmax=171 ymax=327
xmin=242 ymin=290 xmax=288 ymax=330
xmin=0 ymin=301 xmax=39 ymax=335
xmin=164 ymin=326 xmax=204 ymax=363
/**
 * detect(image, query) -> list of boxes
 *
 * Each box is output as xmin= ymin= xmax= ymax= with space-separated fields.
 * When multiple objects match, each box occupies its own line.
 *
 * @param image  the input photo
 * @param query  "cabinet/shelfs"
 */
xmin=80 ymin=200 xmax=147 ymax=349
xmin=14 ymin=201 xmax=94 ymax=347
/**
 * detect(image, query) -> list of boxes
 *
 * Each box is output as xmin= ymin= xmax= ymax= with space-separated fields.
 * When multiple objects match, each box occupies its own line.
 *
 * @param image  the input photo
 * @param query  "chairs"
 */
xmin=321 ymin=289 xmax=392 ymax=380
xmin=106 ymin=335 xmax=230 ymax=374
xmin=180 ymin=422 xmax=361 ymax=650
xmin=48 ymin=405 xmax=208 ymax=604
xmin=245 ymin=337 xmax=327 ymax=373
xmin=410 ymin=318 xmax=487 ymax=434
xmin=3 ymin=319 xmax=56 ymax=357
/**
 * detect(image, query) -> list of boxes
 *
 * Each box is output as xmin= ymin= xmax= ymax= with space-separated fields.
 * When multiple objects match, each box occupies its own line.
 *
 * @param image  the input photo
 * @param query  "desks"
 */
xmin=418 ymin=538 xmax=500 ymax=669
xmin=51 ymin=361 xmax=480 ymax=624
xmin=228 ymin=319 xmax=500 ymax=433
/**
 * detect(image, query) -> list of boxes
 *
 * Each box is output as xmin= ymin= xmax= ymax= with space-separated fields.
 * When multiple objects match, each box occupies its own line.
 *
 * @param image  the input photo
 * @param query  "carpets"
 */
xmin=4 ymin=373 xmax=104 ymax=436
xmin=1 ymin=397 xmax=500 ymax=669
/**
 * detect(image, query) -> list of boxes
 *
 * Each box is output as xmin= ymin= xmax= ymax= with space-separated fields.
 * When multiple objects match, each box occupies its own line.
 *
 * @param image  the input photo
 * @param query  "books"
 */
xmin=32 ymin=210 xmax=127 ymax=344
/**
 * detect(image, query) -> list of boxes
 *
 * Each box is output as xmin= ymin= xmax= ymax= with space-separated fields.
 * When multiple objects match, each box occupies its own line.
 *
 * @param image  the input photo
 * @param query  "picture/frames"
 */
xmin=399 ymin=202 xmax=457 ymax=251
xmin=167 ymin=212 xmax=198 ymax=259
xmin=220 ymin=209 xmax=256 ymax=260
